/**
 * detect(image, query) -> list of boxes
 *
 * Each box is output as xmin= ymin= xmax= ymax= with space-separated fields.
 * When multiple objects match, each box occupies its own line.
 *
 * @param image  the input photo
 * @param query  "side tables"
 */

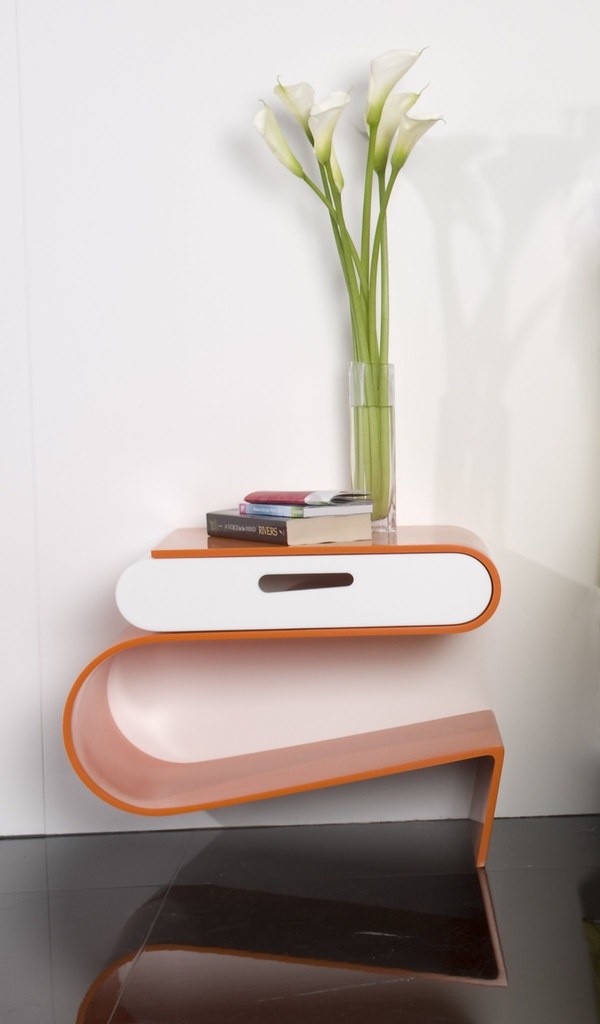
xmin=60 ymin=524 xmax=506 ymax=869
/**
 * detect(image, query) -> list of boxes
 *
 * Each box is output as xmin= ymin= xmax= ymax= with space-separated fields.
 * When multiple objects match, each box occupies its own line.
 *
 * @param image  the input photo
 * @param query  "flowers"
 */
xmin=249 ymin=45 xmax=450 ymax=534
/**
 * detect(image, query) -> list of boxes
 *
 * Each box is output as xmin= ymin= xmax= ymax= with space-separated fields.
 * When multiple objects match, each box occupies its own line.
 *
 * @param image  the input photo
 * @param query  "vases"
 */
xmin=348 ymin=360 xmax=397 ymax=534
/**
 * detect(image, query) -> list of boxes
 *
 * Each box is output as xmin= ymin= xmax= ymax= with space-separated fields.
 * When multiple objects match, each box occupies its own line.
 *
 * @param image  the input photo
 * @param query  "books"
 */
xmin=205 ymin=508 xmax=372 ymax=546
xmin=238 ymin=503 xmax=374 ymax=517
